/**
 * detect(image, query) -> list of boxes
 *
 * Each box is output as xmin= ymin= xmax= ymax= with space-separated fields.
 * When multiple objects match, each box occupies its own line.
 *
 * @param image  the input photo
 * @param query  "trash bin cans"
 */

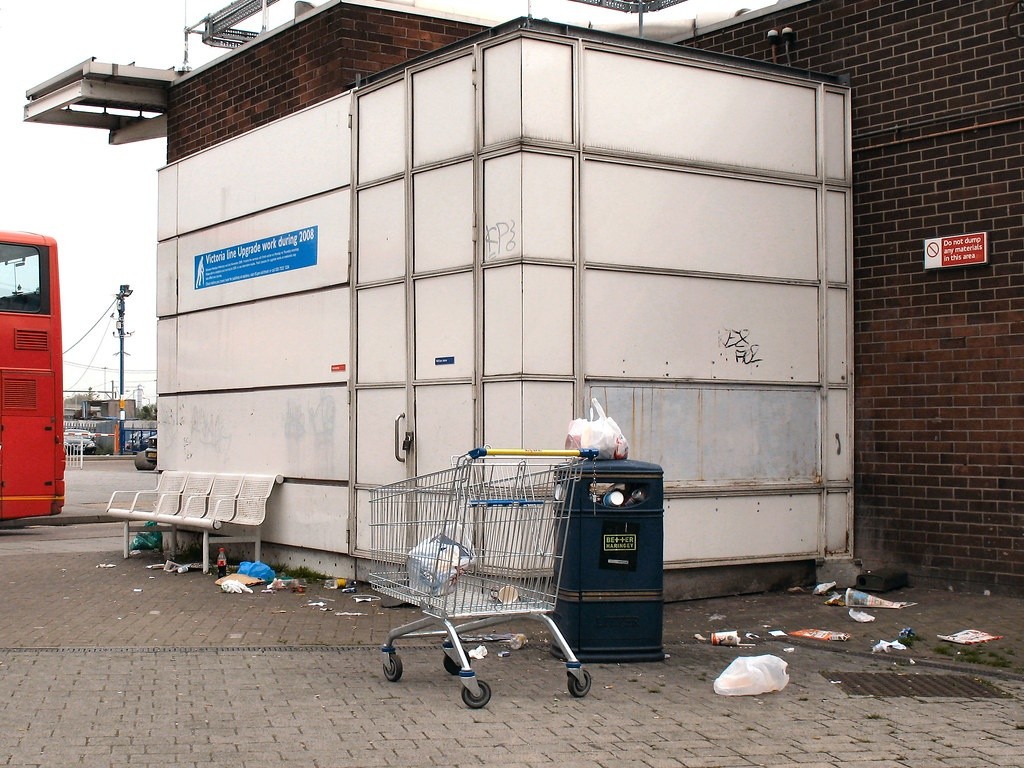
xmin=551 ymin=458 xmax=667 ymax=663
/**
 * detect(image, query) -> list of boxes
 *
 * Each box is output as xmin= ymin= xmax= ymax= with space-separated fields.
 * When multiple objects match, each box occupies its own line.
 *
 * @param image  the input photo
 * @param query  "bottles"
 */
xmin=217 ymin=548 xmax=227 ymax=579
xmin=324 ymin=579 xmax=357 ymax=590
xmin=510 ymin=634 xmax=528 ymax=649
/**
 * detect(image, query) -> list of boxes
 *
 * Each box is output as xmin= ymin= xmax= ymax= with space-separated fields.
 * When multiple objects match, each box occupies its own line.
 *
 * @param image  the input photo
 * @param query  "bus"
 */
xmin=0 ymin=230 xmax=66 ymax=524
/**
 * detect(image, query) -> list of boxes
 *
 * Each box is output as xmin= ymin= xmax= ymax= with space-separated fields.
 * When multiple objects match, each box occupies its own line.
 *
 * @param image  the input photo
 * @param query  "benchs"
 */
xmin=106 ymin=470 xmax=282 ymax=573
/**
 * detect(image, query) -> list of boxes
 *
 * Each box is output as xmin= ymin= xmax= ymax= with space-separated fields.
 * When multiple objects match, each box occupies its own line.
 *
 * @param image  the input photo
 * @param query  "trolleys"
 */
xmin=367 ymin=445 xmax=600 ymax=710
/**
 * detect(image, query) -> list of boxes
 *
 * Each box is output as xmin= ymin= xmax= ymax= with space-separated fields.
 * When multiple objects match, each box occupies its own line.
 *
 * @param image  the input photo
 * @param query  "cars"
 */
xmin=62 ymin=429 xmax=97 ymax=454
xmin=143 ymin=434 xmax=162 ymax=474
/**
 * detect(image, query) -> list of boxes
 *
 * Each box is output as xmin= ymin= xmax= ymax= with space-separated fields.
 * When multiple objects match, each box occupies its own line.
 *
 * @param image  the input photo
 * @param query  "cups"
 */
xmin=603 ymin=492 xmax=624 ymax=508
xmin=711 ymin=631 xmax=740 ymax=646
